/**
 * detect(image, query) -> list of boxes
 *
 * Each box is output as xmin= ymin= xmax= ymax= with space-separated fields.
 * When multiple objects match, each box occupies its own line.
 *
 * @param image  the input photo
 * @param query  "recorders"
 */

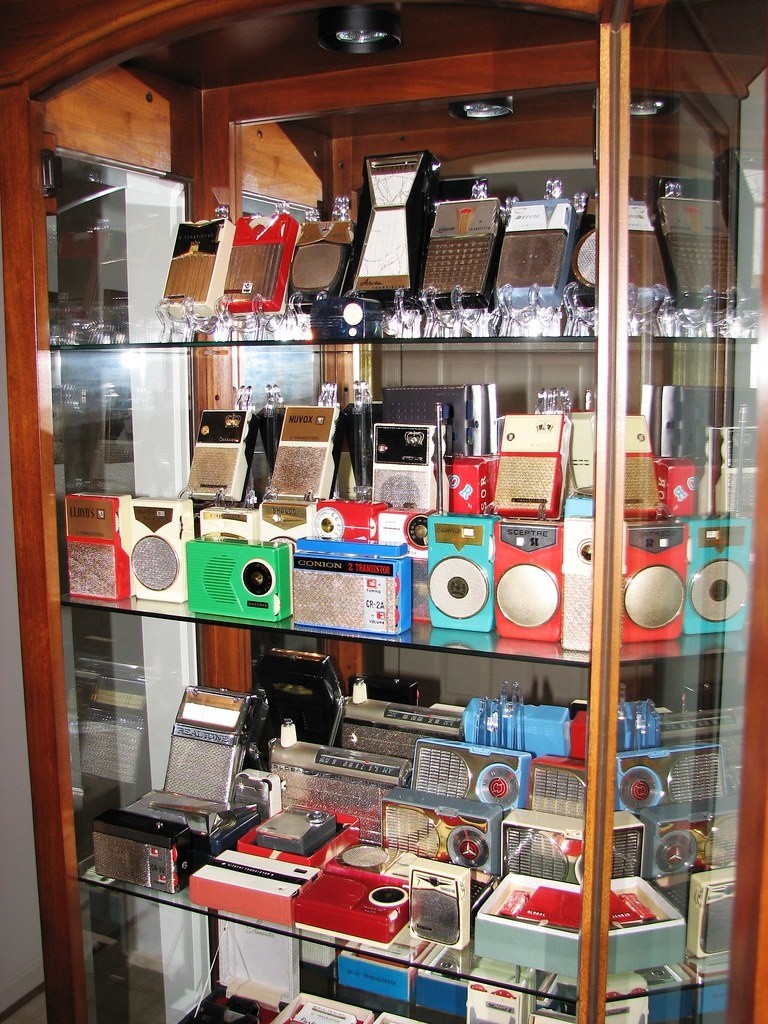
xmin=66 ymin=150 xmax=753 ymax=962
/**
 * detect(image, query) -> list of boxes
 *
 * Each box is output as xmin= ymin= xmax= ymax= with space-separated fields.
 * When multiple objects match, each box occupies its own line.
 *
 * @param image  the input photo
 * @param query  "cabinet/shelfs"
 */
xmin=0 ymin=0 xmax=767 ymax=1024
